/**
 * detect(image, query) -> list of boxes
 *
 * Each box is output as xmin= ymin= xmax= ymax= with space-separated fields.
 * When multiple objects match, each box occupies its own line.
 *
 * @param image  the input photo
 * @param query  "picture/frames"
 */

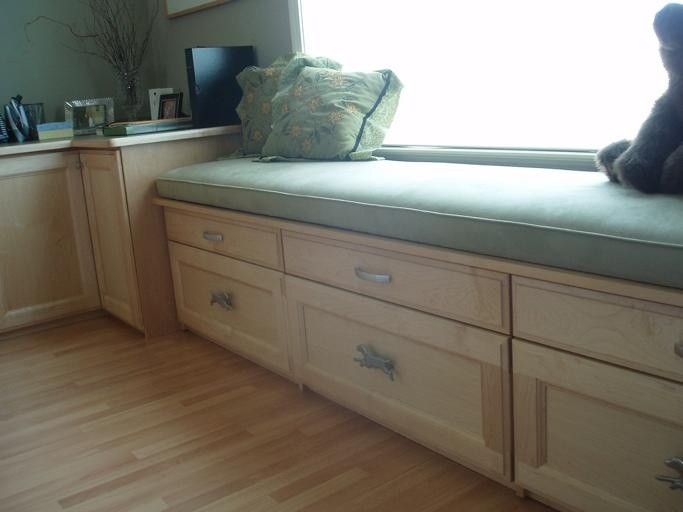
xmin=157 ymin=91 xmax=183 ymax=119
xmin=164 ymin=0 xmax=233 ymax=20
xmin=64 ymin=97 xmax=115 ymax=135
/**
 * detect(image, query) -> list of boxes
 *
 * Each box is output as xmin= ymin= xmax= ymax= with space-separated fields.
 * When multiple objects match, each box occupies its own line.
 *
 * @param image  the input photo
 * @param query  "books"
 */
xmin=36 ymin=120 xmax=73 ymax=131
xmin=37 ymin=128 xmax=74 ymax=141
xmin=103 ymin=117 xmax=193 ymax=136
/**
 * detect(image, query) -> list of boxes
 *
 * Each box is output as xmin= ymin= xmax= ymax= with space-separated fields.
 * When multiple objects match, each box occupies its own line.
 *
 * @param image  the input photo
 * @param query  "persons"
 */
xmin=81 ymin=109 xmax=96 ymax=129
xmin=24 ymin=104 xmax=37 ymax=132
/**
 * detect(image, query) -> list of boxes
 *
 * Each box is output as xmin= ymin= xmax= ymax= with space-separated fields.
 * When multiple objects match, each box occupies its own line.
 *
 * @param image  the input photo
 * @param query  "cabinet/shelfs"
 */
xmin=1 ymin=133 xmax=98 ymax=330
xmin=152 ymin=133 xmax=682 ymax=512
xmin=76 ymin=132 xmax=239 ymax=341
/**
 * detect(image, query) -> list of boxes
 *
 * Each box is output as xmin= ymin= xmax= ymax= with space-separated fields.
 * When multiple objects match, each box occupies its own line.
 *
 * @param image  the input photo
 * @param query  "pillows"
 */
xmin=234 ymin=51 xmax=403 ymax=161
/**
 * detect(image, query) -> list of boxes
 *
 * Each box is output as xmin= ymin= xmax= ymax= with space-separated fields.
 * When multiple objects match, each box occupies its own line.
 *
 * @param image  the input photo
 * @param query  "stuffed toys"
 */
xmin=592 ymin=1 xmax=683 ymax=197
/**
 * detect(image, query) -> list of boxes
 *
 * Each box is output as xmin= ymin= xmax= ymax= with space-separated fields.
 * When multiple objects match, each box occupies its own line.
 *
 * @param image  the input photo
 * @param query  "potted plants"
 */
xmin=24 ymin=0 xmax=160 ymax=123
